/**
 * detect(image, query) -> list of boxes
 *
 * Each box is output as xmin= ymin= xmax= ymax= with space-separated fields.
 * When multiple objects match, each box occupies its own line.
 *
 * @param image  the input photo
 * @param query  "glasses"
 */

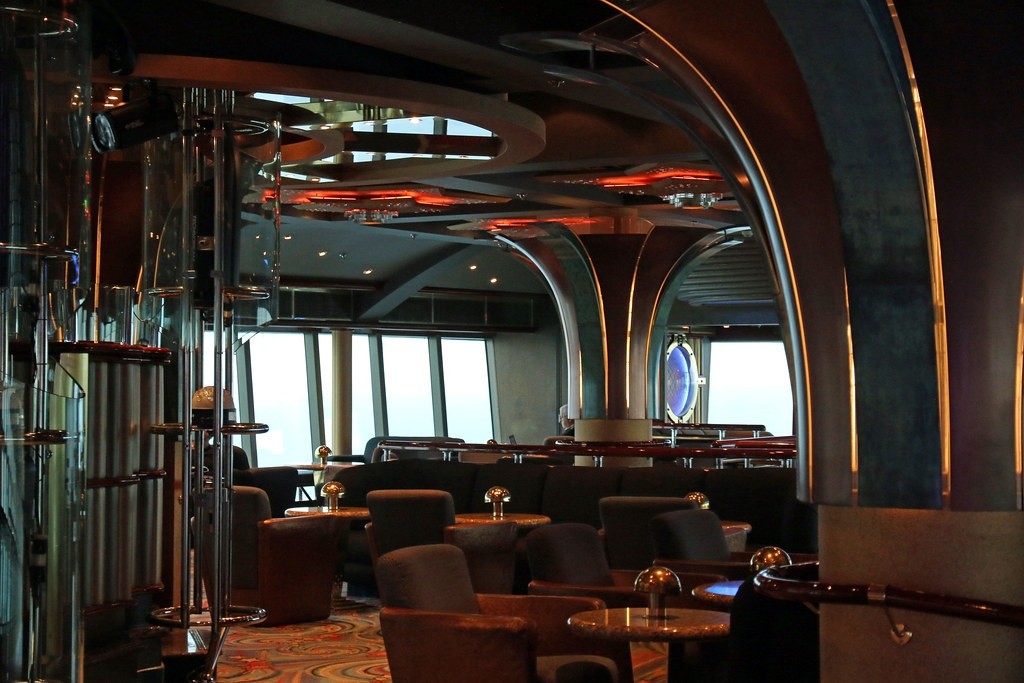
xmin=558 ymin=414 xmax=569 ymax=423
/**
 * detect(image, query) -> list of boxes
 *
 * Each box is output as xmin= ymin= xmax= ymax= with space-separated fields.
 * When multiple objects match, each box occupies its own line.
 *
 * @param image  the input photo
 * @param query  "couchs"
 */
xmin=524 ymin=523 xmax=729 ymax=610
xmin=364 ymin=489 xmax=520 ymax=596
xmin=231 ymin=486 xmax=338 ymax=626
xmin=652 ymin=509 xmax=820 ymax=580
xmin=596 ymin=496 xmax=701 ymax=569
xmin=376 ymin=542 xmax=612 ymax=683
xmin=323 ymin=435 xmax=797 ymax=523
xmin=197 ymin=444 xmax=299 ymax=521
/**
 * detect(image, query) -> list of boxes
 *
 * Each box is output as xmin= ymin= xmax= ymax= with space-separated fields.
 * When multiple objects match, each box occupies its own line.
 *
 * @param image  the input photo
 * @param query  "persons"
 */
xmin=558 ymin=404 xmax=578 ymax=447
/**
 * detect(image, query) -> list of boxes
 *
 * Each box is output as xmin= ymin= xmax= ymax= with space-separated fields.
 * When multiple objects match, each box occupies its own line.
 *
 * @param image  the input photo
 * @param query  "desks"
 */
xmin=567 ymin=607 xmax=731 ymax=683
xmin=284 ymin=504 xmax=370 ymax=615
xmin=719 ymin=519 xmax=753 ymax=553
xmin=295 ymin=461 xmax=365 ymax=488
xmin=690 ymin=577 xmax=749 ymax=606
xmin=455 ymin=512 xmax=552 ymax=535
